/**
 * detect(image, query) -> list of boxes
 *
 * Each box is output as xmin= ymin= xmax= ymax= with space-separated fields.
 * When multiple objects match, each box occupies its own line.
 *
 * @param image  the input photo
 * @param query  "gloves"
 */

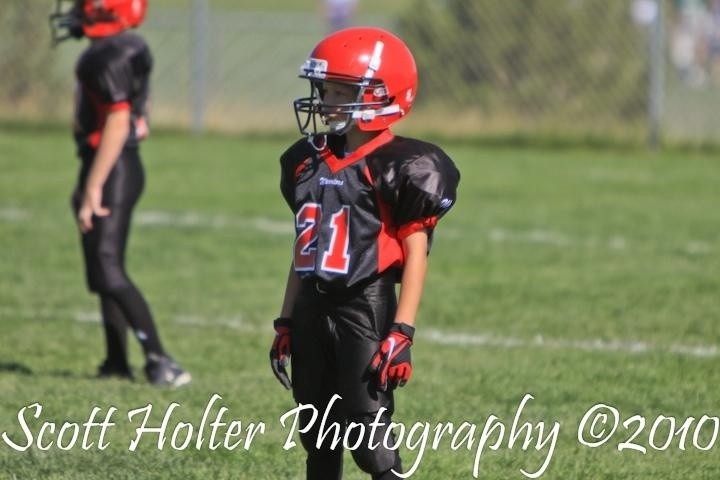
xmin=369 ymin=323 xmax=413 ymax=390
xmin=269 ymin=318 xmax=292 ymax=389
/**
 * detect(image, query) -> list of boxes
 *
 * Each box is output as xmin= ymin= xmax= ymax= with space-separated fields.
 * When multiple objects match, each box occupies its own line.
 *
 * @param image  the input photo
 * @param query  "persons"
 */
xmin=268 ymin=26 xmax=463 ymax=480
xmin=45 ymin=0 xmax=192 ymax=390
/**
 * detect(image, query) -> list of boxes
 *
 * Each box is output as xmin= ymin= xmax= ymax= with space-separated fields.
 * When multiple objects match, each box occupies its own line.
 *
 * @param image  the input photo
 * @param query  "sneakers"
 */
xmin=92 ymin=360 xmax=134 ymax=381
xmin=144 ymin=355 xmax=192 ymax=387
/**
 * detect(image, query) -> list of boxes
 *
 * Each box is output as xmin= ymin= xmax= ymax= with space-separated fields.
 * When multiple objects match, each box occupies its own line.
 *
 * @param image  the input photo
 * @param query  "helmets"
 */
xmin=295 ymin=26 xmax=418 ymax=144
xmin=47 ymin=1 xmax=149 ymax=50
